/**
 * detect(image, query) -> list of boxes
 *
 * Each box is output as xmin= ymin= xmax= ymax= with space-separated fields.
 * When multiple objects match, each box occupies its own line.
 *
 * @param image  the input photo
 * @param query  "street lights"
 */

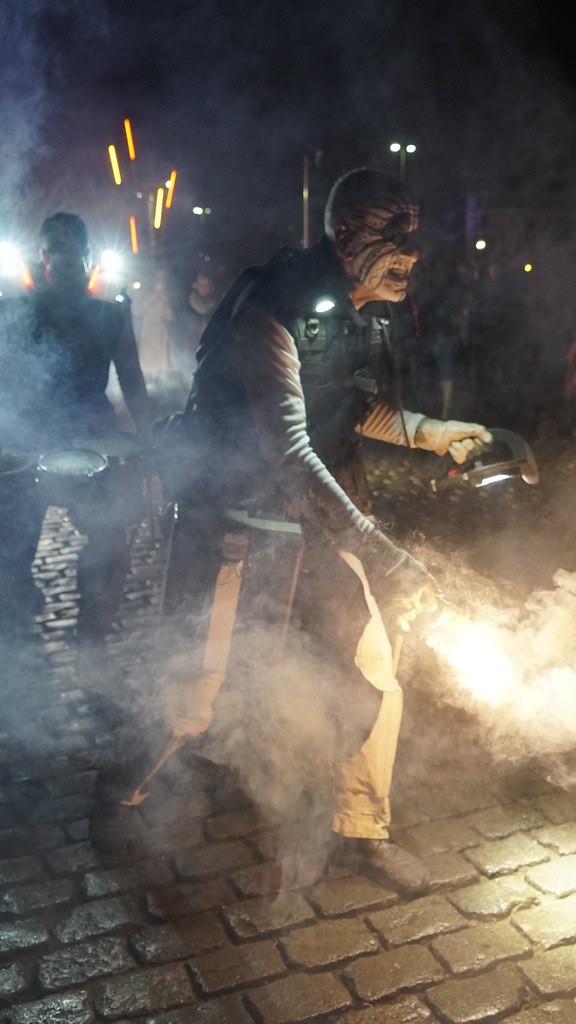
xmin=391 ymin=142 xmax=417 ymax=185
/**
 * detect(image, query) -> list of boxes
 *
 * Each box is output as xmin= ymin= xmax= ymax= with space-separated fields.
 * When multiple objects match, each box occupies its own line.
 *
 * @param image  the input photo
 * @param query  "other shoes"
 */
xmin=76 ymin=651 xmax=129 ymax=685
xmin=89 ymin=770 xmax=124 ymax=859
xmin=323 ymin=830 xmax=430 ymax=893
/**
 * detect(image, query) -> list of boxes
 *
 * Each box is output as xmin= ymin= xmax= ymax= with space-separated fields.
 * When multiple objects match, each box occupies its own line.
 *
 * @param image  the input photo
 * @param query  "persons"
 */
xmin=1 ymin=166 xmax=495 ymax=893
